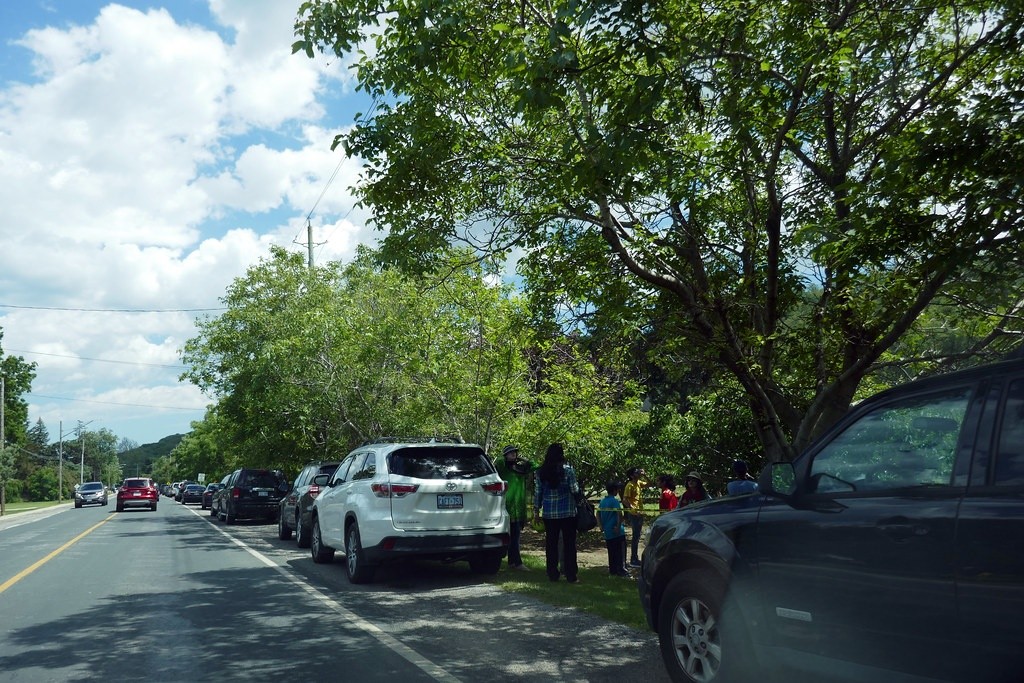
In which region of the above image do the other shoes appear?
[629,556,642,568]
[624,572,633,579]
[573,579,581,584]
[556,574,567,581]
[508,563,515,568]
[515,564,530,572]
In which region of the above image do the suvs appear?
[175,482,184,501]
[638,347,1024,683]
[217,467,290,525]
[177,481,199,502]
[154,483,159,501]
[309,437,510,585]
[117,477,157,511]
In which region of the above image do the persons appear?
[676,471,712,511]
[656,473,678,514]
[726,460,758,495]
[495,445,540,571]
[557,528,565,576]
[534,442,583,583]
[623,467,654,567]
[596,480,631,578]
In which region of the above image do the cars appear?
[162,486,170,495]
[160,484,167,492]
[201,483,219,510]
[277,460,342,548]
[166,488,172,497]
[182,485,207,505]
[211,474,231,516]
[75,482,108,508]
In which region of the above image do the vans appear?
[171,483,179,494]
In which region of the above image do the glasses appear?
[632,470,639,475]
[688,479,696,482]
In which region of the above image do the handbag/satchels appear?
[576,494,597,530]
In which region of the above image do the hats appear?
[606,481,623,491]
[503,445,518,455]
[687,471,701,480]
